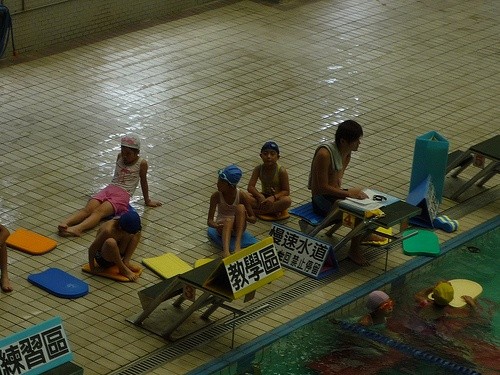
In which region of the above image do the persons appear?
[0,225,12,292]
[207,165,256,258]
[307,121,386,267]
[360,290,392,328]
[58,133,162,237]
[247,141,291,219]
[88,211,141,281]
[415,280,475,313]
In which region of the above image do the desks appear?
[444,135,500,200]
[308,201,422,253]
[136,257,234,337]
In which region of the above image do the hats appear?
[432,280,455,306]
[119,211,142,232]
[367,290,390,311]
[260,141,281,154]
[120,133,141,150]
[220,165,243,182]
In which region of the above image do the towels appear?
[307,142,344,190]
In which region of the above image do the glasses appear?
[217,169,235,187]
[262,142,278,148]
[377,300,394,310]
[436,280,451,286]
